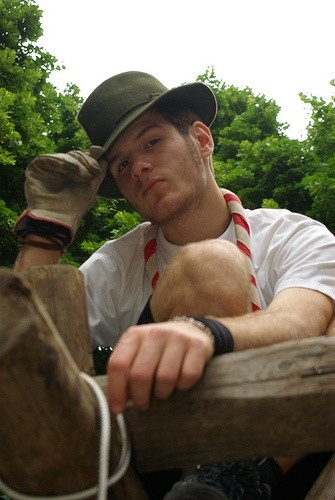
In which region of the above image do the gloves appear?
[13,145,107,248]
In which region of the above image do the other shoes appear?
[164,455,272,500]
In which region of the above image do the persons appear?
[14,71,335,500]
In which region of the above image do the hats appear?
[77,71,217,198]
